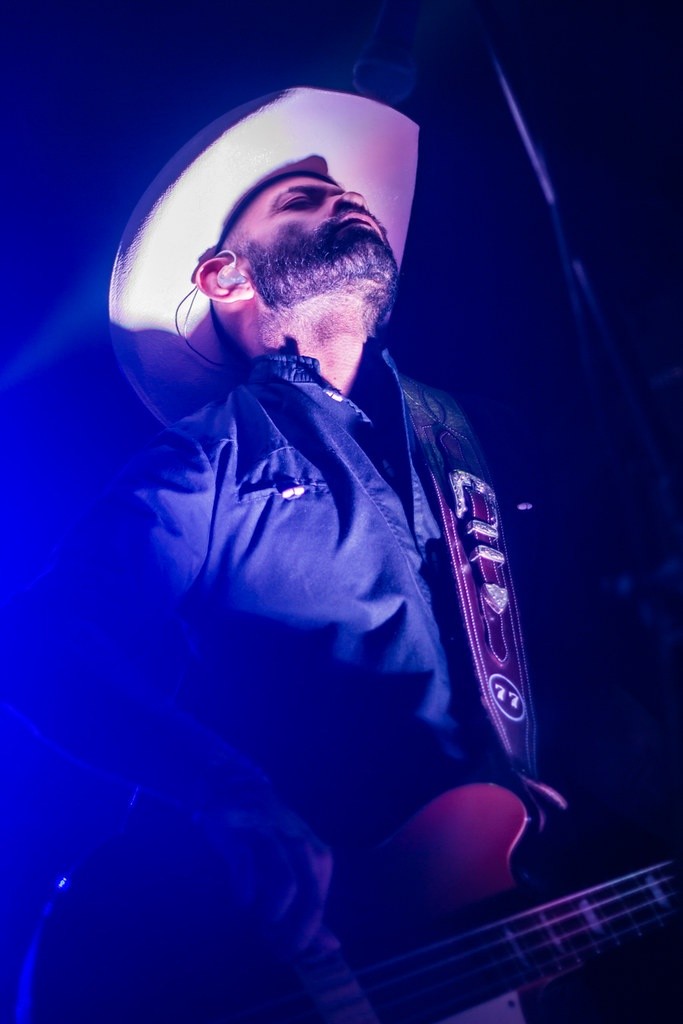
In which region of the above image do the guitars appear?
[15,782,682,1023]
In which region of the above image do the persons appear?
[0,83,574,1024]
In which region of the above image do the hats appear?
[104,88,422,425]
